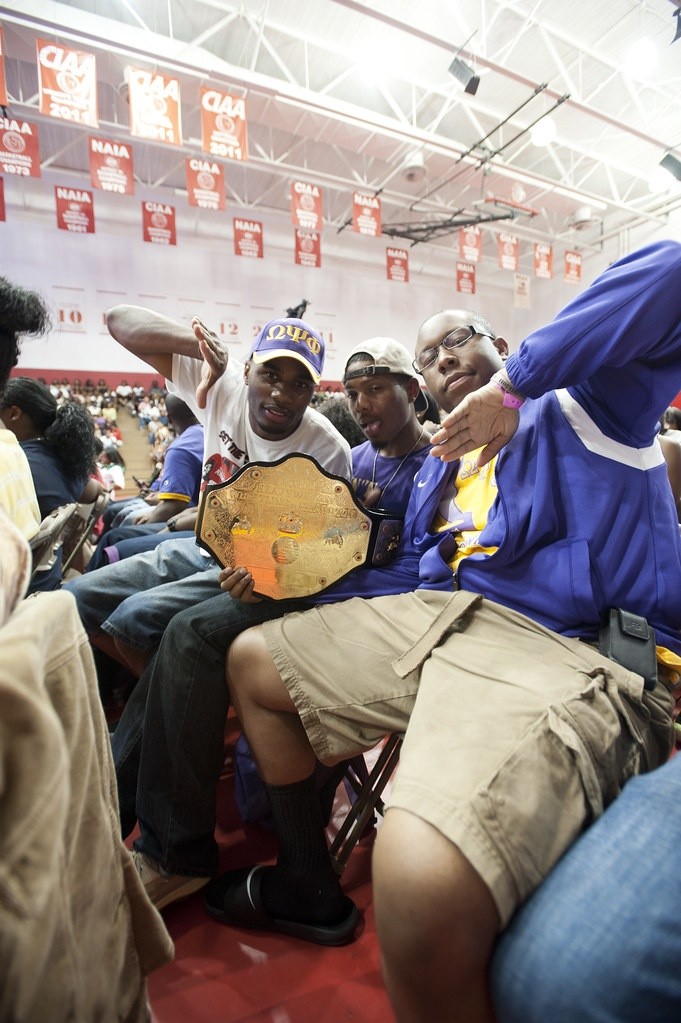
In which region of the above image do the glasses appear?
[412,324,496,374]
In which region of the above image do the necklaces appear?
[372,425,425,507]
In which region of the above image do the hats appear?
[342,338,429,412]
[248,318,325,385]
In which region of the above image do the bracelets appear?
[493,371,527,401]
[490,380,523,409]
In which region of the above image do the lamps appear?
[660,154,681,186]
[400,57,481,182]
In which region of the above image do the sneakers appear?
[132,851,208,910]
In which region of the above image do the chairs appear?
[26,479,114,591]
[327,732,407,875]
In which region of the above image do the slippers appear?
[203,866,357,946]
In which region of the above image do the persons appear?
[202,235,681,1023]
[0,274,681,911]
[489,745,681,1023]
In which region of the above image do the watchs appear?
[167,517,178,532]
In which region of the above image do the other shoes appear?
[111,676,136,699]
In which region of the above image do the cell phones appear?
[131,476,142,490]
